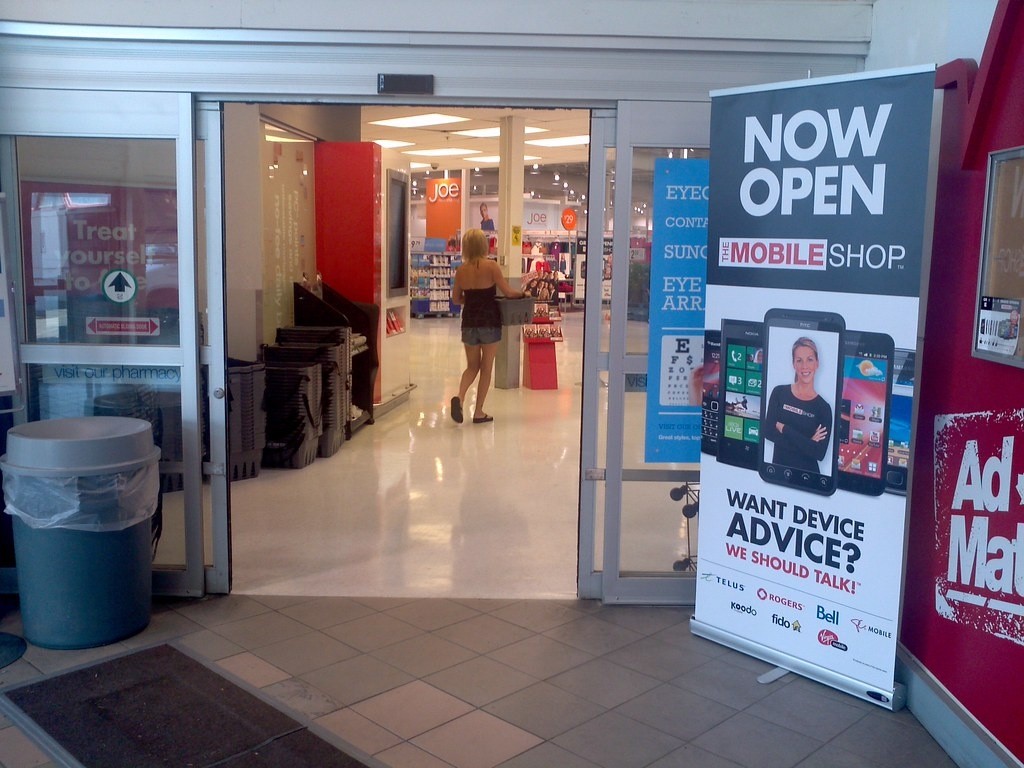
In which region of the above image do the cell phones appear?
[700,308,918,497]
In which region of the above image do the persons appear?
[765,337,833,473]
[451,229,532,423]
[480,203,495,231]
[527,271,558,305]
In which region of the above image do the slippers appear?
[450,397,463,423]
[473,414,493,423]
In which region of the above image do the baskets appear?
[90,326,352,492]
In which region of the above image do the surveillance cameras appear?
[431,163,440,170]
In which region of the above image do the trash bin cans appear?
[0,415,162,650]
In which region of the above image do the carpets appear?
[0,639,394,768]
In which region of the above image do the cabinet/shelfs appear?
[298,283,378,438]
[411,250,461,318]
